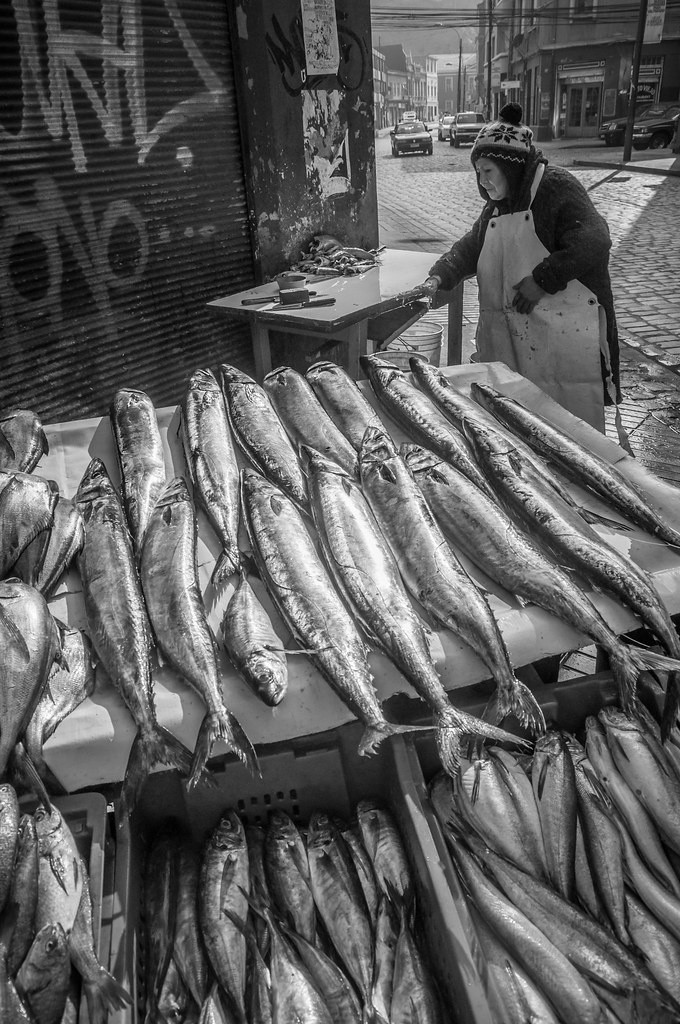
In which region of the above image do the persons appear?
[420,100,622,437]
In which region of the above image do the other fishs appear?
[0,351,679,1024]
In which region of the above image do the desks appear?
[205,248,477,381]
[25,360,680,792]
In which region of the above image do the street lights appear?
[446,63,466,112]
[433,23,462,114]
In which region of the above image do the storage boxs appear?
[19,664,680,1024]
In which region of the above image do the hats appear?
[470,103,533,216]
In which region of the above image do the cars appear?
[437,116,456,142]
[599,101,680,148]
[389,121,434,158]
[632,104,680,150]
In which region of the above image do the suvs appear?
[449,111,488,149]
